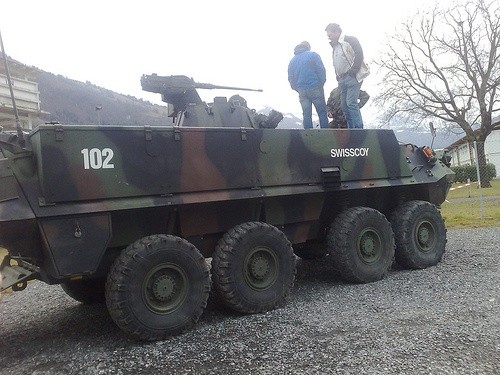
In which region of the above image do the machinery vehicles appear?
[0,72,458,343]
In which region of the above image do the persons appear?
[324,23,371,129]
[441,148,452,168]
[287,40,329,129]
[326,76,370,128]
